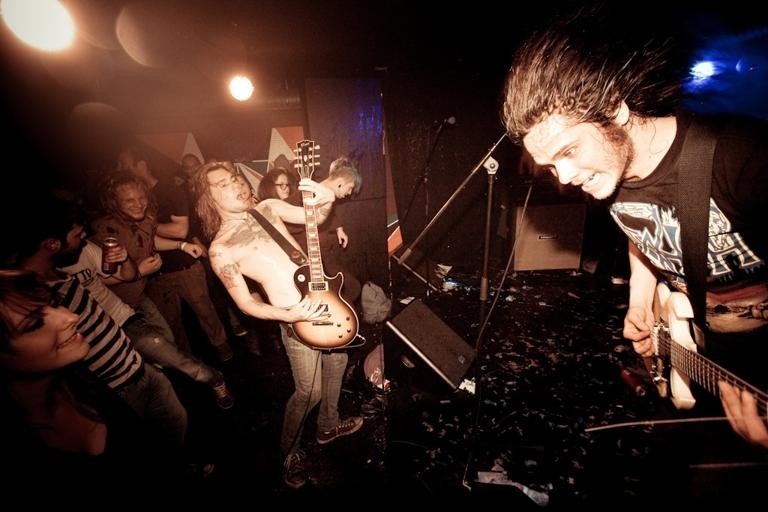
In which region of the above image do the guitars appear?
[640,283,766,423]
[292,139,359,350]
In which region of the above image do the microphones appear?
[425,116,456,131]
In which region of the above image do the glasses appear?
[274,184,288,190]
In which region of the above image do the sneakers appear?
[232,325,247,336]
[219,342,233,361]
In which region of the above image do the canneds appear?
[102,237,120,274]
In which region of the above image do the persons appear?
[502,31,768,448]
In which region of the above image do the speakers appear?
[513,204,586,272]
[386,298,476,390]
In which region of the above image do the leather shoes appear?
[212,372,233,409]
[284,450,306,487]
[316,416,363,444]
